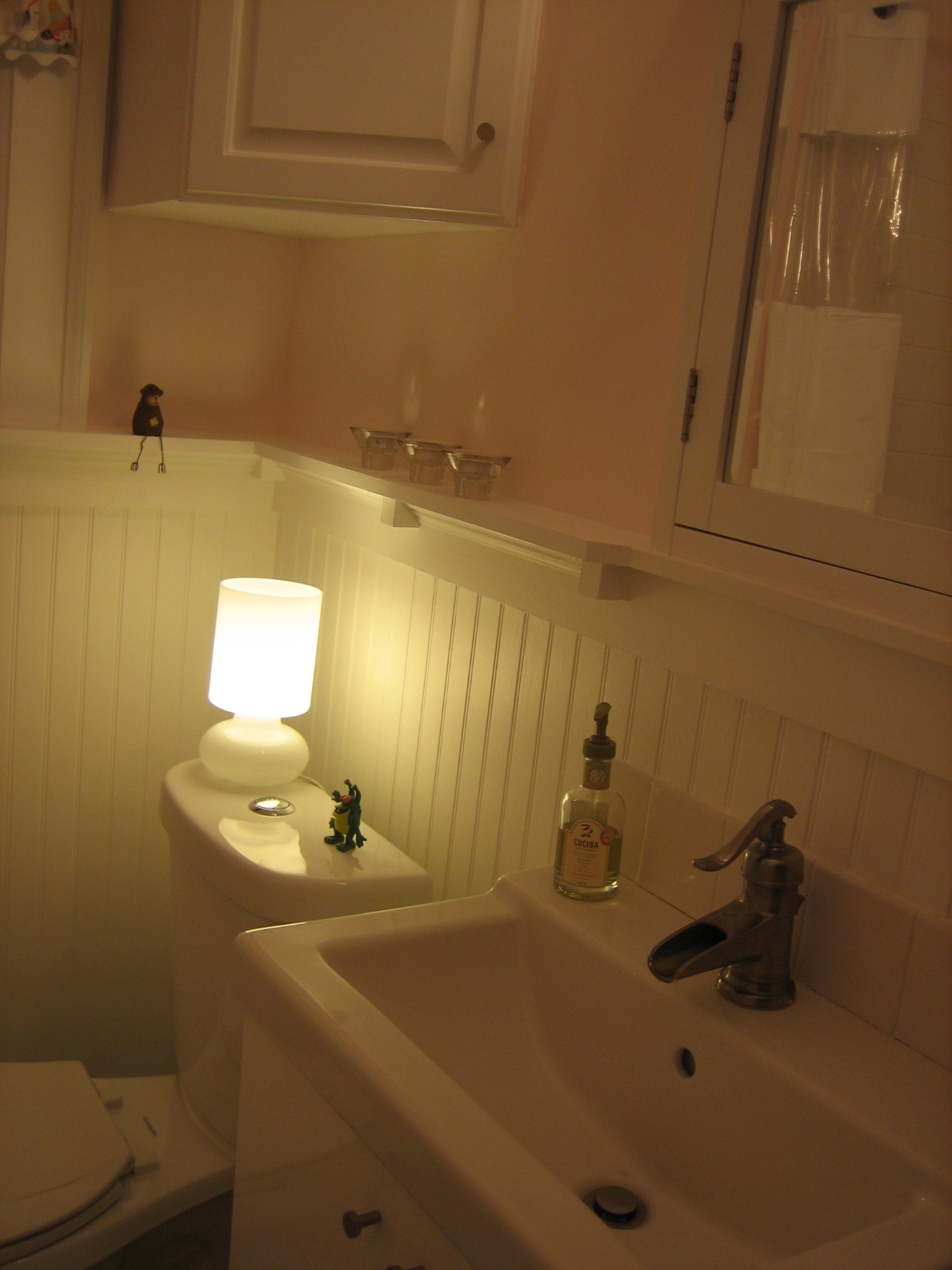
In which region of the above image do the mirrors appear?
[722,1,952,530]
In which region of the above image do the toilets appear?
[1,756,433,1269]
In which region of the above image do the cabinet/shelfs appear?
[105,1,544,240]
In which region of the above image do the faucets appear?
[646,798,806,1011]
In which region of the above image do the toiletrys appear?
[551,702,627,901]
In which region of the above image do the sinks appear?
[234,875,952,1270]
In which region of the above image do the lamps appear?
[199,579,325,787]
